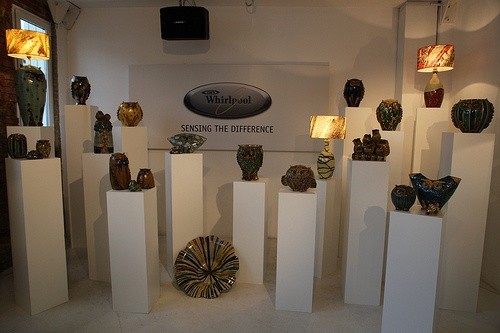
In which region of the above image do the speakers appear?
[159,6,210,40]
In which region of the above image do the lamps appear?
[6,29,50,126]
[309,115,346,180]
[416,45,455,108]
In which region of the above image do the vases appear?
[237,144,263,181]
[70,75,91,105]
[117,102,143,126]
[36,139,51,159]
[451,98,495,133]
